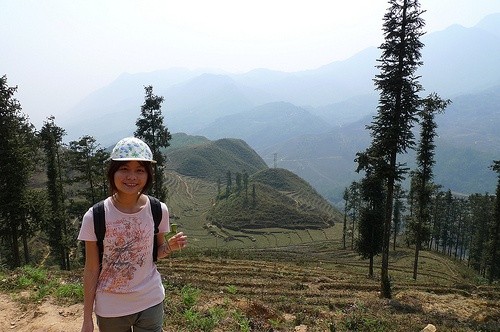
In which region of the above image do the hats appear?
[103,138,159,165]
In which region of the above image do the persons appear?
[76,136,187,332]
